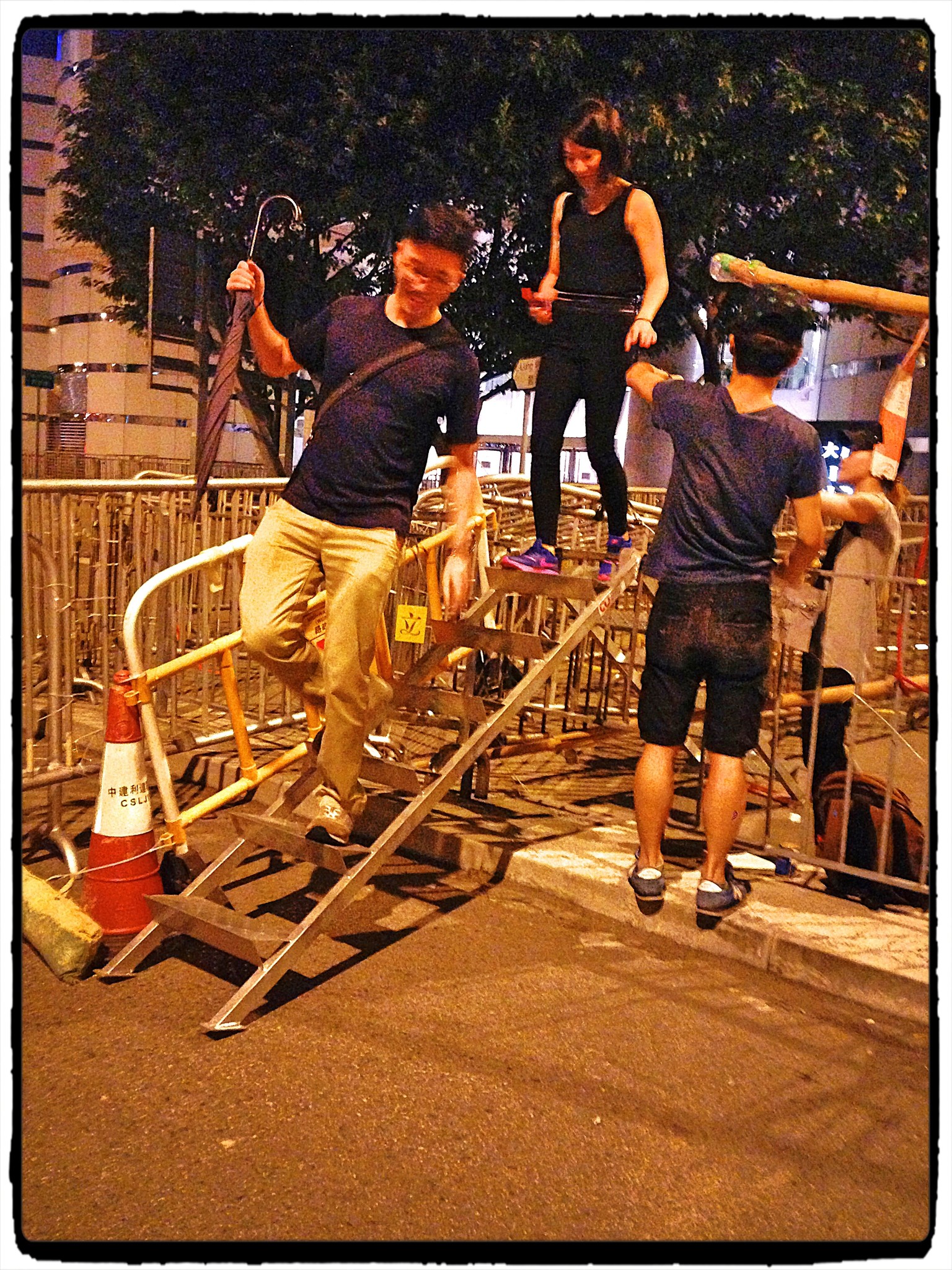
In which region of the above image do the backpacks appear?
[815,771,927,907]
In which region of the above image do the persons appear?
[223,200,489,845]
[781,431,916,848]
[615,310,828,920]
[491,100,671,587]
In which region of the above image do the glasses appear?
[395,258,461,292]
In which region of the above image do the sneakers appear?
[501,538,559,576]
[305,795,353,846]
[696,872,752,917]
[628,861,666,900]
[597,536,632,581]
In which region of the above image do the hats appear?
[837,423,912,460]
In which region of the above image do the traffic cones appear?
[78,672,169,961]
